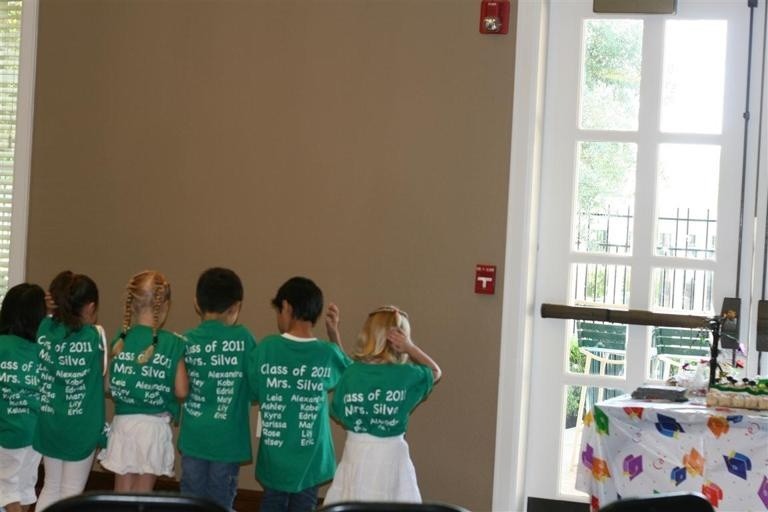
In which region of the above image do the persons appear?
[324,306,441,505]
[1,285,48,512]
[95,272,187,495]
[182,267,261,505]
[252,277,349,510]
[34,270,114,512]
